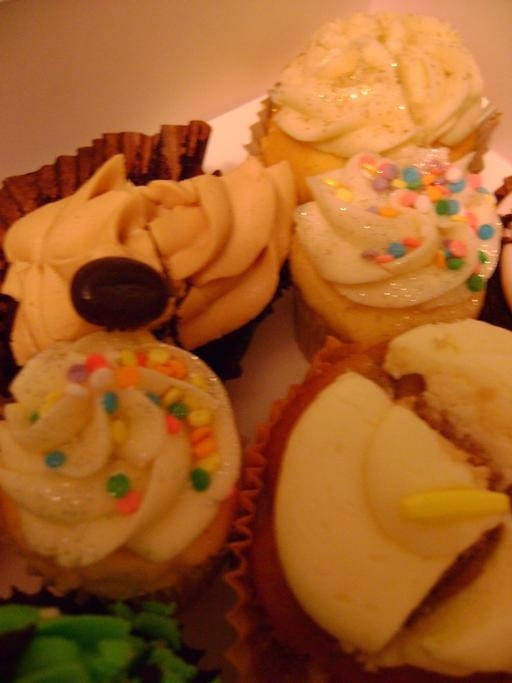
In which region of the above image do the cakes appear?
[227,319,511,679]
[0,598,217,681]
[1,151,295,373]
[1,332,242,596]
[293,146,502,341]
[247,14,500,201]
